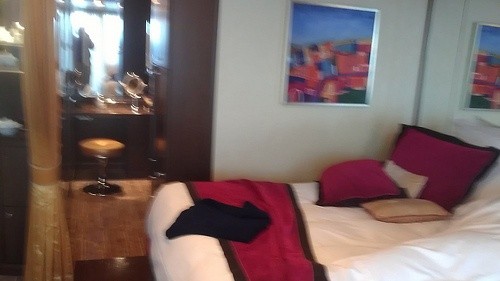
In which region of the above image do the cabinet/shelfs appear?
[75,102,154,168]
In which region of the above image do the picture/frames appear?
[281,0,381,109]
[459,23,500,111]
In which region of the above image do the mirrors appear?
[55,0,128,97]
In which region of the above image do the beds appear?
[140,116,500,281]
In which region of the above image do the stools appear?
[82,139,124,196]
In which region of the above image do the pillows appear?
[315,127,500,224]
